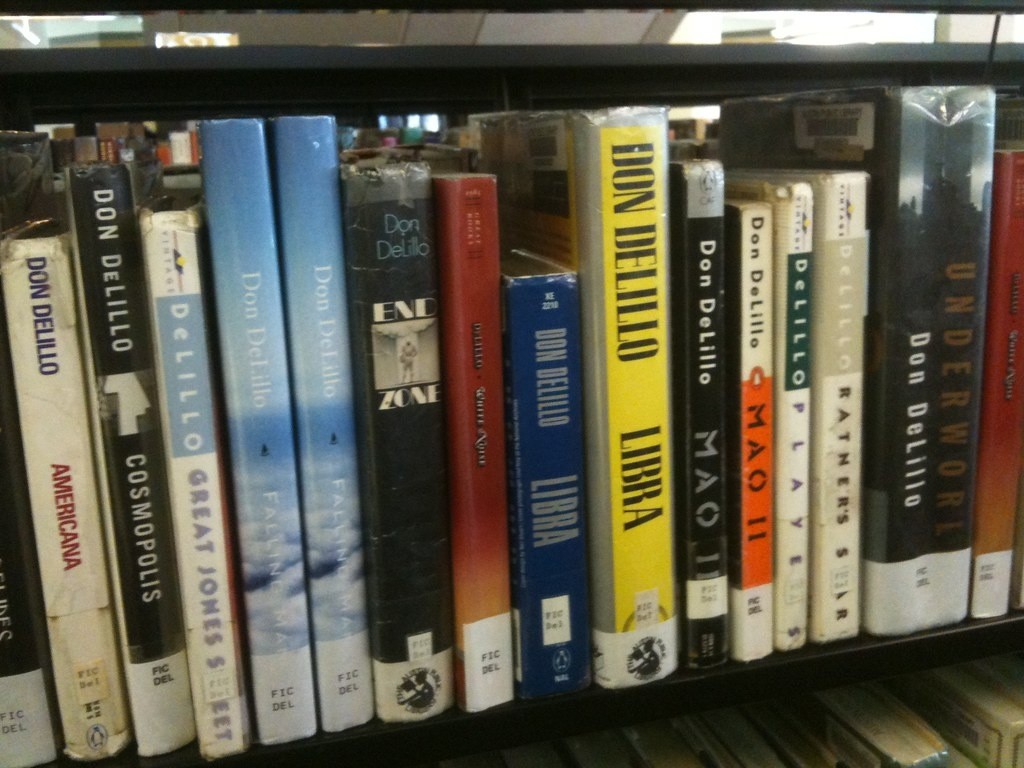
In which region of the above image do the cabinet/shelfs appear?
[0,0,1024,768]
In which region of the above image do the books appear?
[722,197,775,661]
[500,247,592,697]
[141,188,251,763]
[0,84,1024,768]
